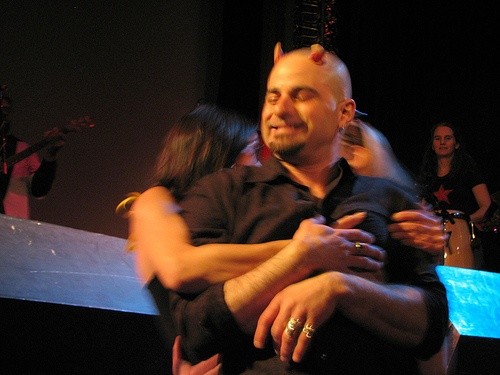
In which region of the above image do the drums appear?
[433,209,476,269]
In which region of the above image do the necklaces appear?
[437,161,452,173]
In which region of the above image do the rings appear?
[352,243,362,254]
[303,325,316,338]
[281,317,303,338]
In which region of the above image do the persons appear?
[1,83,66,224]
[417,125,492,269]
[165,41,450,375]
[125,103,448,374]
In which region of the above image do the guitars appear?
[0,113,97,174]
